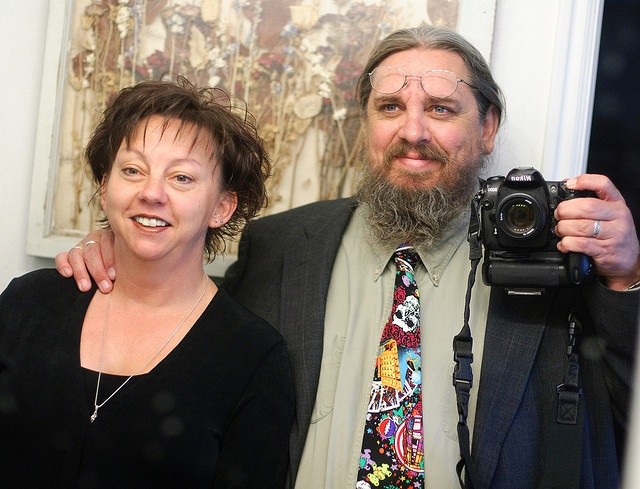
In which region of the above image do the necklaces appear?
[89,273,211,423]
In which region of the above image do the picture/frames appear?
[25,2,495,277]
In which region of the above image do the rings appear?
[591,219,600,238]
[67,246,83,253]
[84,240,97,248]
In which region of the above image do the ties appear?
[356,242,424,489]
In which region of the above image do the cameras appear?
[467,166,594,305]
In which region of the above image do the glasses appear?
[365,65,478,98]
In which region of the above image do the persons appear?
[52,27,639,485]
[0,76,293,489]
